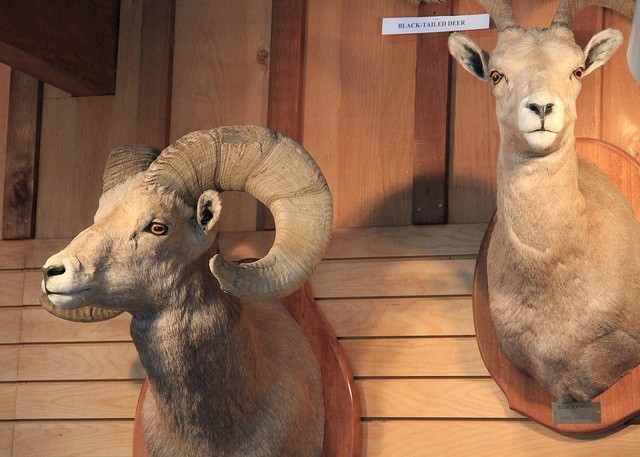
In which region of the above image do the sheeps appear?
[41,124,333,456]
[446,0,640,405]
